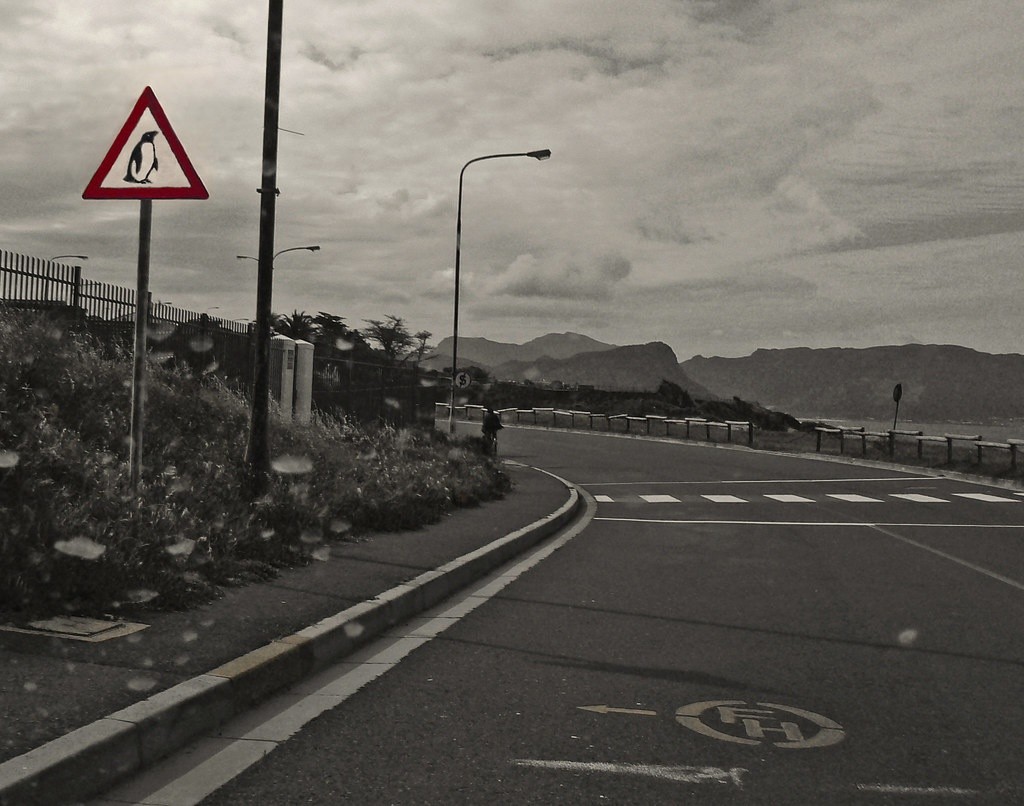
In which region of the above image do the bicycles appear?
[484,431,499,455]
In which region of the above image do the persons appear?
[481,408,504,453]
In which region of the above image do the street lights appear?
[448,148,551,433]
[205,306,219,313]
[236,245,321,261]
[158,301,172,305]
[234,318,249,322]
[48,255,89,264]
[77,283,102,286]
